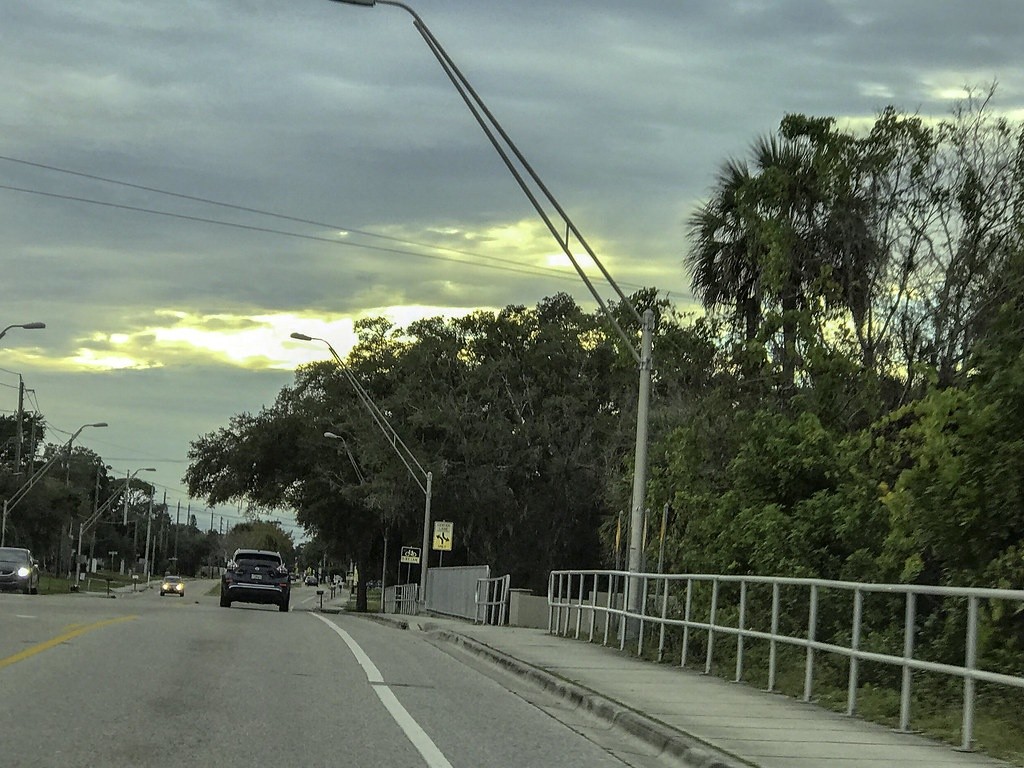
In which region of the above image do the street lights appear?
[2,423,111,550]
[74,467,156,588]
[321,431,392,614]
[290,330,434,609]
[330,1,657,641]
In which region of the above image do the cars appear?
[290,573,296,581]
[219,548,290,613]
[0,547,40,595]
[304,576,319,587]
[160,576,185,597]
